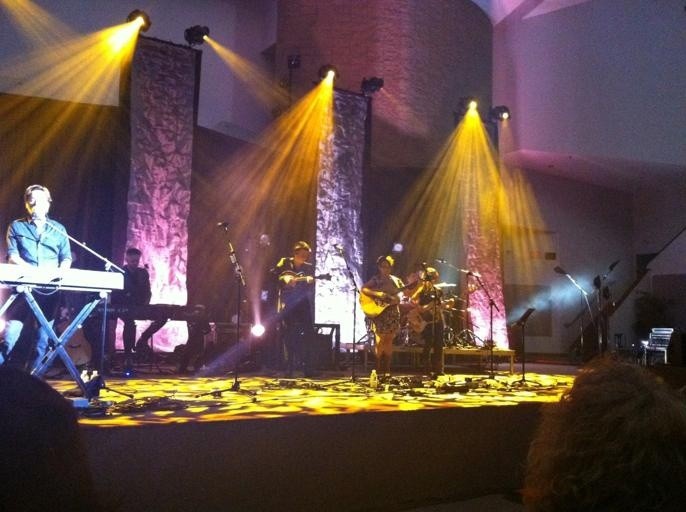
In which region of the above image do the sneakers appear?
[304,373,319,377]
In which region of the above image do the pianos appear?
[87,304,197,320]
[0,263,124,294]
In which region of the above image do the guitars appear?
[407,288,478,333]
[278,271,332,289]
[359,280,423,317]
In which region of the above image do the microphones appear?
[217,221,229,227]
[31,210,39,219]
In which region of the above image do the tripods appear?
[450,264,485,346]
[194,258,256,399]
[321,284,367,388]
[509,332,543,386]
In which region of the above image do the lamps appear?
[360,77,384,95]
[127,9,151,32]
[185,25,210,45]
[319,63,340,84]
[491,104,511,122]
[459,95,479,114]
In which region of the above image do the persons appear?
[522,354,686,511]
[361,256,455,376]
[109,248,167,355]
[271,242,314,379]
[1,367,89,512]
[0,185,72,373]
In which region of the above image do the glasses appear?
[131,260,139,263]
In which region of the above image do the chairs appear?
[640,328,674,366]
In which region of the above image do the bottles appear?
[370,370,377,388]
